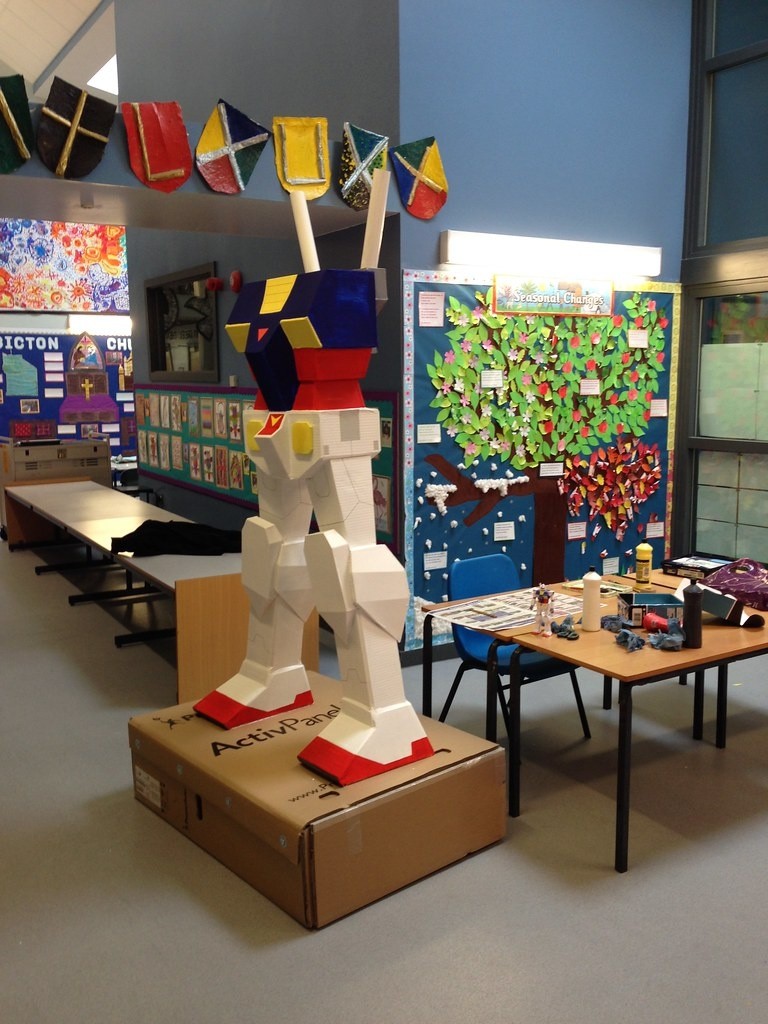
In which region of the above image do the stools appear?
[116,485,153,503]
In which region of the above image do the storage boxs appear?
[617,592,684,626]
[129,672,509,929]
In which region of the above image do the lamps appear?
[440,229,662,276]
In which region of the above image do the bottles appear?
[635,538,654,588]
[682,578,702,648]
[582,566,601,632]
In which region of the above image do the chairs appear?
[438,554,591,764]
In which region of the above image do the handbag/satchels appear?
[696,557,768,611]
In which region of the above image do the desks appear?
[422,569,768,872]
[3,476,319,705]
